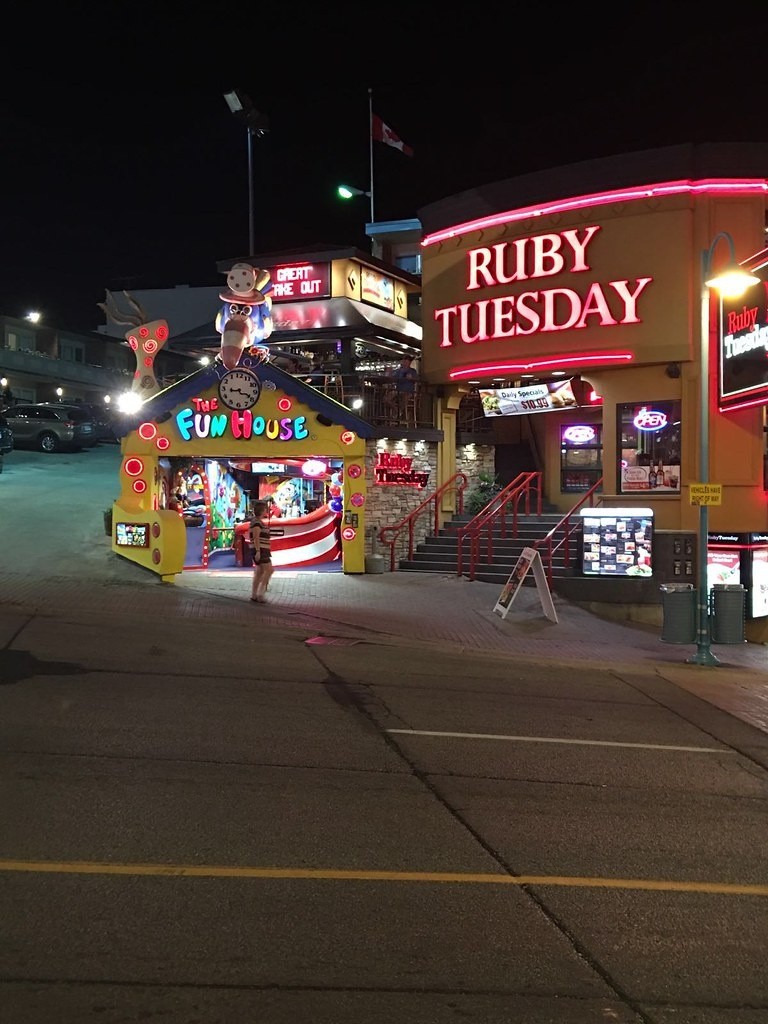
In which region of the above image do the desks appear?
[359,376,392,425]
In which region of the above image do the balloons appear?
[331,473,341,486]
[329,484,341,497]
[328,499,342,513]
[338,471,343,483]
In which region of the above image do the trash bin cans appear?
[659,583,698,644]
[710,584,748,644]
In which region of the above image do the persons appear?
[663,446,680,466]
[286,357,301,374]
[303,355,324,387]
[382,355,418,419]
[249,500,274,604]
[263,496,281,519]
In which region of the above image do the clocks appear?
[218,368,262,411]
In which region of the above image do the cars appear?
[76,403,127,445]
[0,415,13,455]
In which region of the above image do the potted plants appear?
[467,471,513,516]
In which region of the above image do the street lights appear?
[223,89,254,255]
[684,230,761,667]
[337,185,374,224]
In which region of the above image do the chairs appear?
[389,382,418,429]
[324,375,362,416]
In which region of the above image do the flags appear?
[372,113,414,158]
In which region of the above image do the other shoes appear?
[256,601,267,604]
[250,598,258,602]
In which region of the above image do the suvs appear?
[0,404,94,453]
